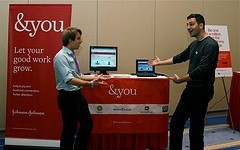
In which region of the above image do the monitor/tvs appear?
[89,46,117,71]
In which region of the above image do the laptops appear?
[136,59,157,77]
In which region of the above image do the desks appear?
[227,71,240,130]
[80,72,171,149]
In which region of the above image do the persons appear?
[53,26,110,150]
[147,13,219,150]
